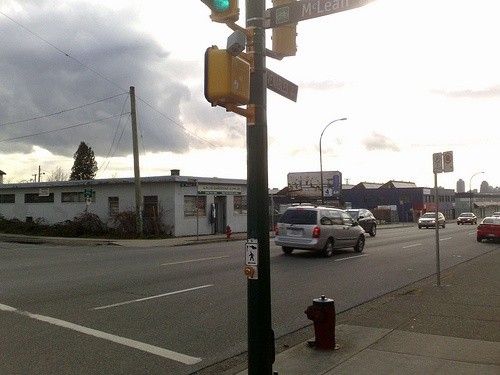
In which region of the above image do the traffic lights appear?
[208,0,239,23]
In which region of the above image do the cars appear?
[274,205,366,258]
[476,217,500,242]
[344,209,377,237]
[418,212,446,229]
[457,213,477,225]
[491,212,500,217]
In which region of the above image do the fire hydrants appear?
[304,295,336,350]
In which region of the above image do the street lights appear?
[470,171,485,213]
[319,118,348,205]
